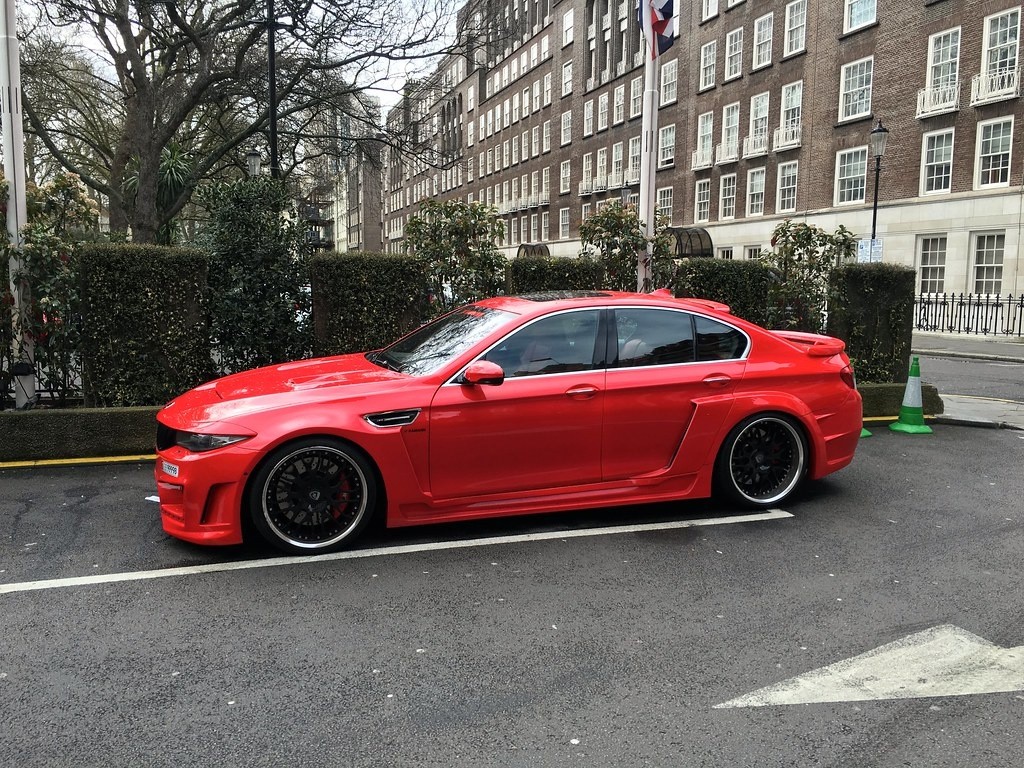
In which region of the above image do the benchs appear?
[619,339,693,365]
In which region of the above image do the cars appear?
[153,288,862,558]
[428,284,490,314]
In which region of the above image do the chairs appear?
[570,324,596,364]
[519,317,571,372]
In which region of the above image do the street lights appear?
[857,118,890,263]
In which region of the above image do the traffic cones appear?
[888,356,934,434]
[849,358,872,438]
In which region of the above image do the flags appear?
[639,0,674,61]
